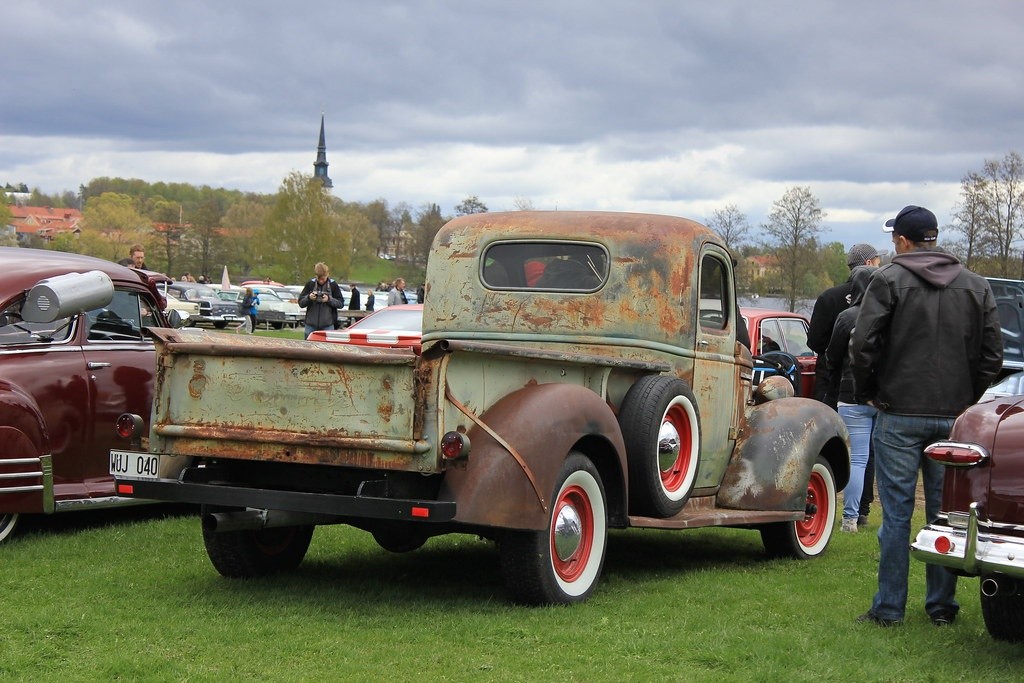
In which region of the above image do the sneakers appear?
[839,515,859,533]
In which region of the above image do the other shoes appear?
[856,609,902,628]
[857,515,867,525]
[932,611,954,626]
[236,328,239,334]
[248,333,253,334]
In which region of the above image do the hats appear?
[253,289,259,294]
[847,244,886,270]
[883,205,938,242]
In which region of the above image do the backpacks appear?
[752,351,802,398]
[307,281,337,322]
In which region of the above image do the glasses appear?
[134,256,146,260]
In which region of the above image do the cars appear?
[1,209,1024,648]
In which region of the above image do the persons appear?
[848,205,1005,626]
[109,244,261,335]
[536,247,608,289]
[344,277,426,331]
[299,262,346,342]
[806,243,886,532]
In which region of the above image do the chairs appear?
[485,257,511,286]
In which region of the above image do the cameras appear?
[314,290,325,302]
